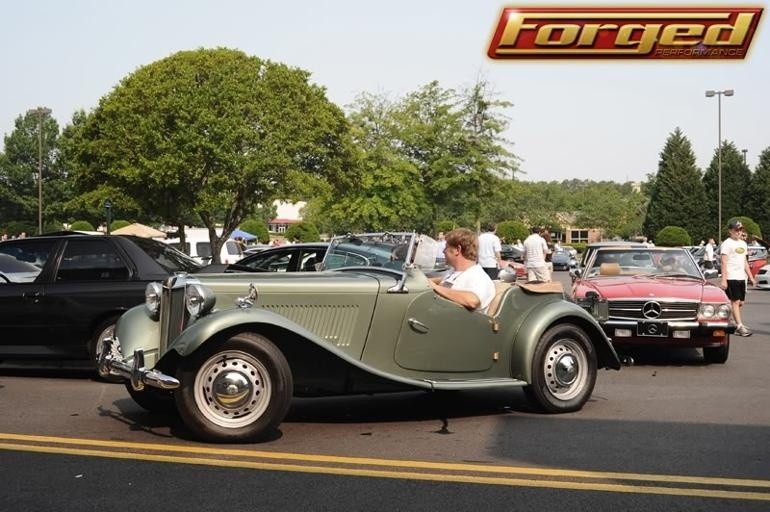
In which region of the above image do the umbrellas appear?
[109,223,166,238]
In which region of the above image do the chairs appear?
[600,263,621,275]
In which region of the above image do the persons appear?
[0,232,26,242]
[234,234,303,254]
[430,222,749,315]
[719,222,756,337]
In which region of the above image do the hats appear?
[728,220,744,231]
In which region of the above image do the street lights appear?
[30,108,51,236]
[103,197,115,236]
[705,90,734,244]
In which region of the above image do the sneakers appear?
[734,325,753,338]
